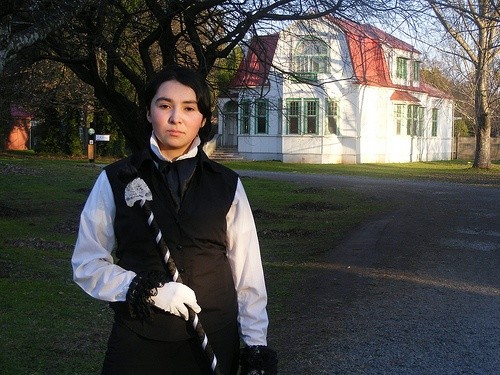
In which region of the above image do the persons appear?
[71,64,280,375]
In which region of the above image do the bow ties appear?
[149,147,199,213]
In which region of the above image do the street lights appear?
[30,121,38,150]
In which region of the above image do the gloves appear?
[125,265,201,321]
[238,345,279,375]
